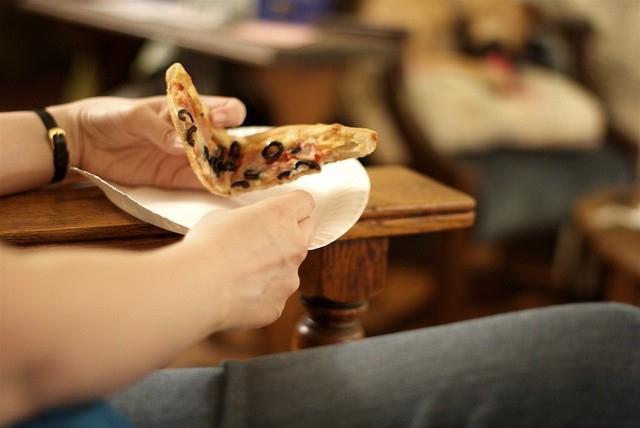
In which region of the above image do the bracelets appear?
[35,103,68,186]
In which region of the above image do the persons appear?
[1,95,621,347]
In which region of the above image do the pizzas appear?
[164,63,378,198]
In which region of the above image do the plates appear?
[64,125,372,253]
